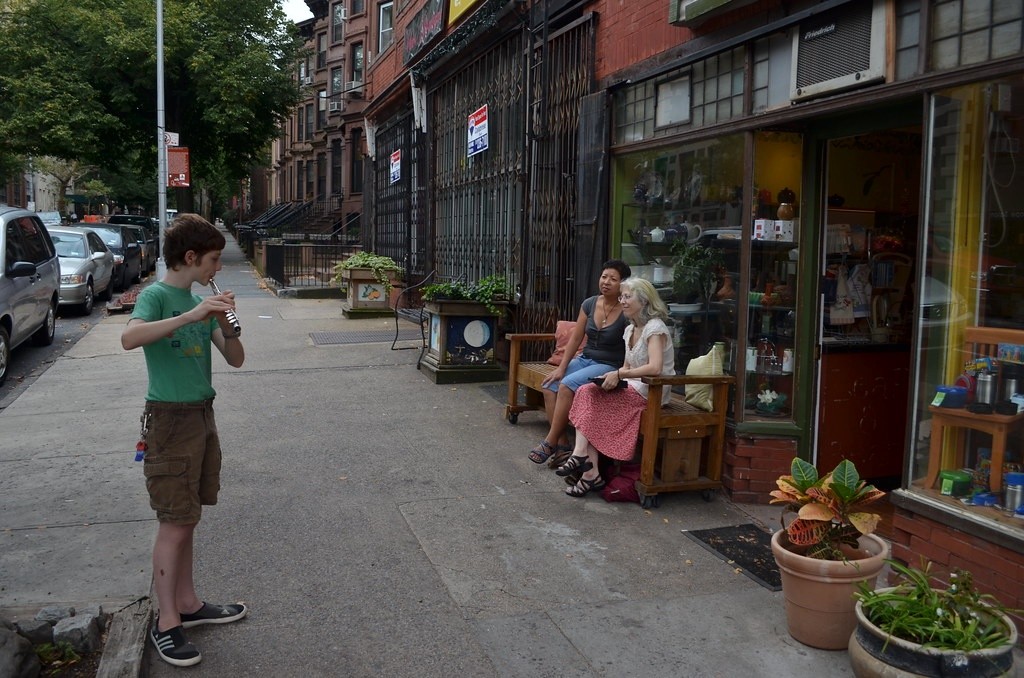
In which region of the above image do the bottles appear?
[759,188,772,203]
[776,203,793,220]
[778,187,796,203]
[760,283,776,306]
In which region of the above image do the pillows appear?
[547,320,588,366]
[683,340,725,412]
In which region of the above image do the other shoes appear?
[565,474,586,486]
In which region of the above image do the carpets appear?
[681,523,782,592]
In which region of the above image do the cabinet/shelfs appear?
[654,238,799,420]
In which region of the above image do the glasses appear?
[617,294,640,302]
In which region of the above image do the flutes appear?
[208,275,242,334]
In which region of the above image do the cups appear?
[782,348,794,372]
[1006,484,1022,510]
[972,373,1019,405]
[746,347,758,371]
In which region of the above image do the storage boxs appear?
[754,220,795,242]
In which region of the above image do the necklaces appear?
[602,301,619,323]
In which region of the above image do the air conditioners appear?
[305,77,313,84]
[341,8,347,21]
[328,102,340,112]
[345,80,361,93]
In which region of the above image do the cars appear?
[35,210,66,226]
[68,213,174,292]
[824,226,1024,352]
[46,226,115,317]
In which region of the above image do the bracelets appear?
[618,370,620,380]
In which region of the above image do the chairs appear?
[924,326,1024,507]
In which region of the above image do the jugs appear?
[649,221,703,244]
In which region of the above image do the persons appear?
[528,259,630,469]
[70,211,77,223]
[121,215,247,665]
[555,277,676,498]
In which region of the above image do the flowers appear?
[851,553,1024,678]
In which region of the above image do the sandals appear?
[528,440,558,464]
[556,455,594,477]
[548,445,573,469]
[565,473,606,497]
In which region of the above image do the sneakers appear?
[178,601,248,627]
[149,615,201,667]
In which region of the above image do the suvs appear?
[0,202,61,386]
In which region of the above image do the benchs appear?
[502,332,734,509]
[390,270,468,369]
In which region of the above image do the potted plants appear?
[746,381,789,417]
[331,251,406,319]
[768,457,889,650]
[418,273,514,384]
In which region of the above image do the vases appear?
[760,275,796,307]
[715,273,736,301]
[848,586,1018,678]
